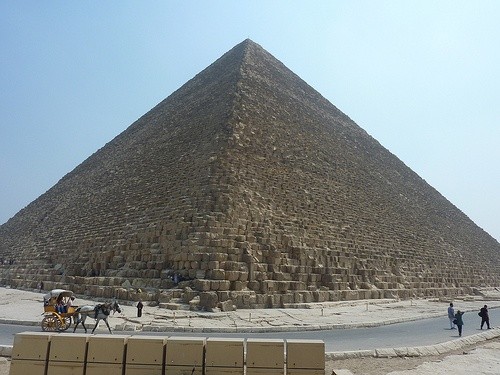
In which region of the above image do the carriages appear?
[39,289,122,334]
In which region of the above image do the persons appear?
[454,310,464,337]
[137,301,143,317]
[53,297,67,314]
[67,296,81,325]
[480,304,491,329]
[448,302,456,329]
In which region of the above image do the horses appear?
[71,298,123,335]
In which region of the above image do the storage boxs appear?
[8,331,327,375]
